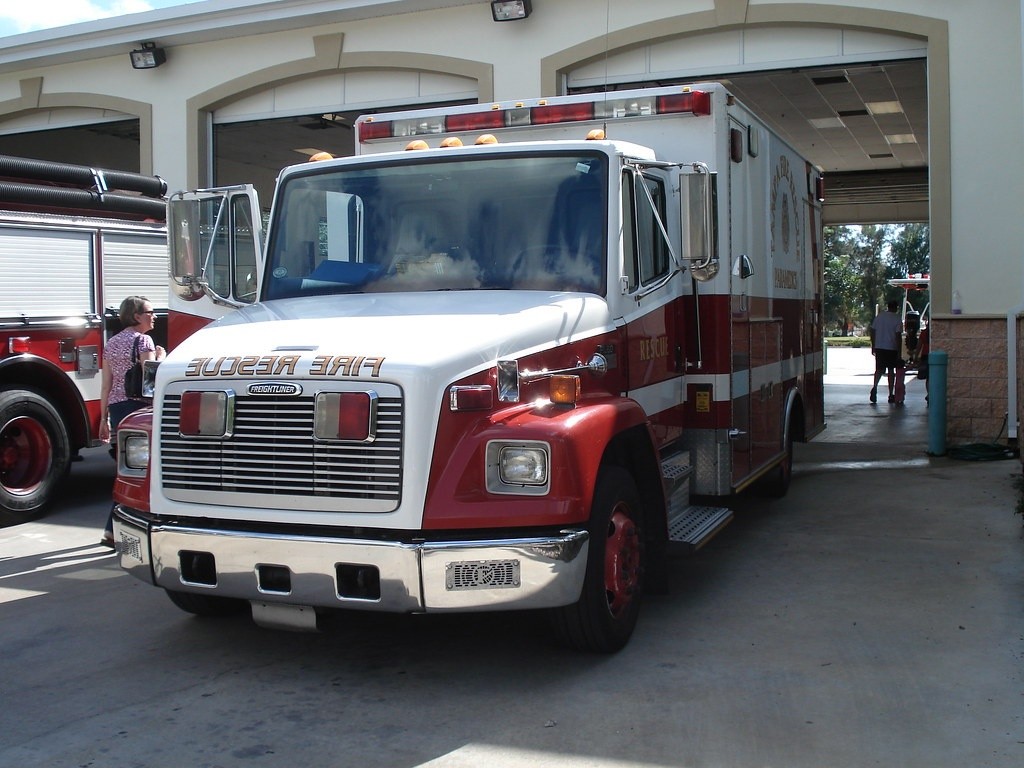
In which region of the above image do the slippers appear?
[99,538,114,549]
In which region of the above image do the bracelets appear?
[100,418,107,420]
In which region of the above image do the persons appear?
[870,301,929,406]
[99,295,165,548]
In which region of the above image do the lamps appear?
[490,0,531,21]
[129,40,167,69]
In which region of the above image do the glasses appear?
[138,311,153,315]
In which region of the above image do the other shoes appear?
[888,395,896,403]
[870,387,876,403]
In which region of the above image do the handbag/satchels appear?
[125,335,152,399]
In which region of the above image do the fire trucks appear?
[873,272,929,371]
[111,80,827,656]
[0,155,173,518]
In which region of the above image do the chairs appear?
[389,206,459,271]
